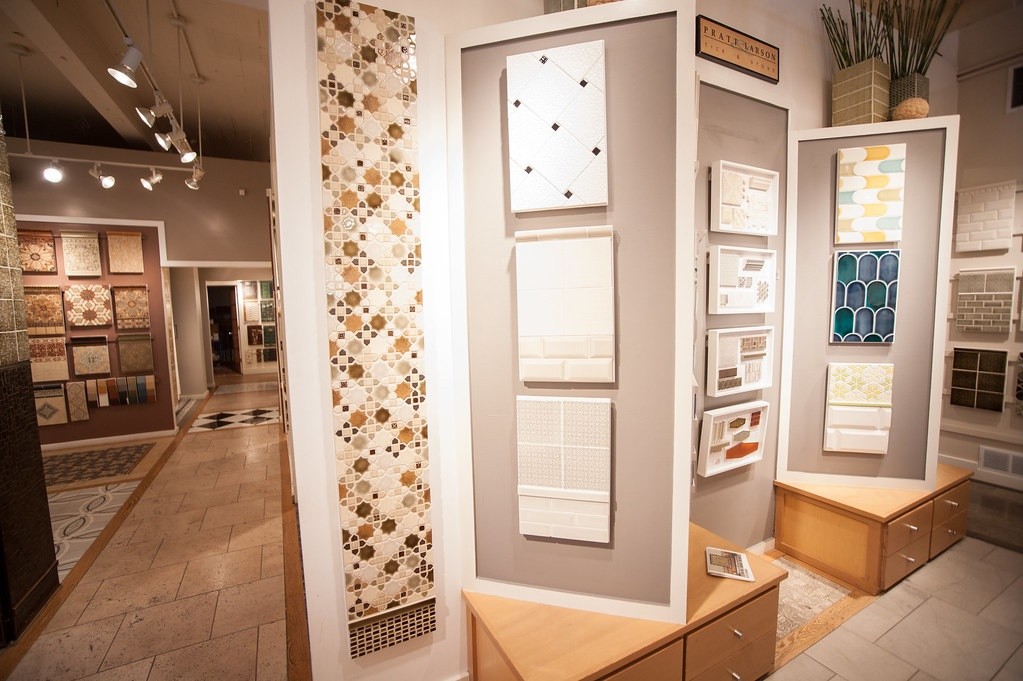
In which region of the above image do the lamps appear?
[42,37,203,191]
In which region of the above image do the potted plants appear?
[817,1,963,128]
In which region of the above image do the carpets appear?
[42,442,158,487]
[760,550,878,680]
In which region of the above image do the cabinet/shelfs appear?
[465,521,788,681]
[773,463,976,595]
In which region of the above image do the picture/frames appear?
[706,325,774,398]
[696,14,779,85]
[708,245,776,315]
[696,400,770,478]
[711,160,780,237]
